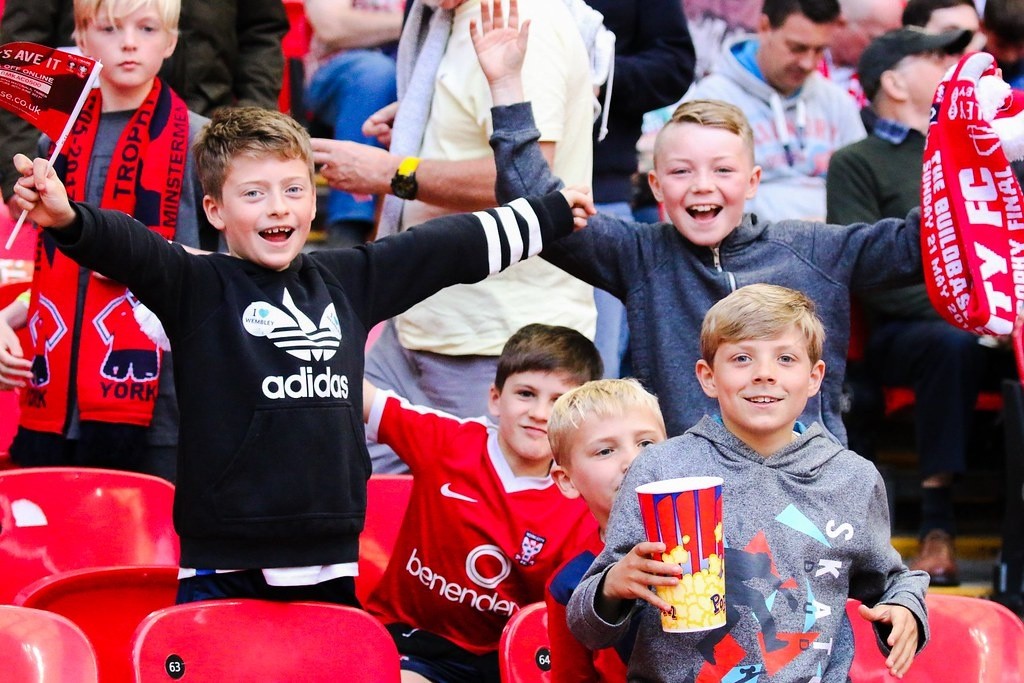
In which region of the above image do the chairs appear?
[0,467,1024,683]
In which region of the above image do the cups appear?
[635,477,727,633]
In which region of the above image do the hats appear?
[856,28,973,101]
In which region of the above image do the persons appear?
[0,0,1024,619]
[564,283,931,683]
[364,324,608,683]
[12,106,599,610]
[550,377,669,683]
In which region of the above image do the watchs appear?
[390,157,422,201]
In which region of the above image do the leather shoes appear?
[909,528,959,587]
